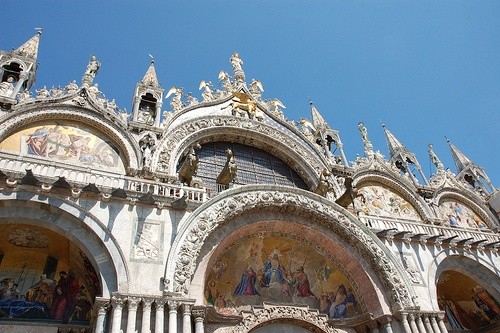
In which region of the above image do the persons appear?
[164,51,328,152]
[331,140,467,194]
[1,45,163,144]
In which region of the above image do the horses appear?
[314,167,338,199]
[335,176,366,210]
[216,148,238,191]
[178,142,201,186]
